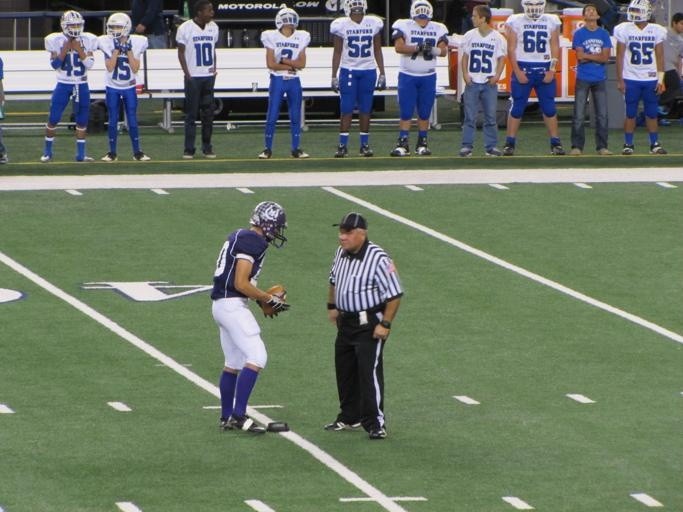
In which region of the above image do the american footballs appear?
[259,286,287,315]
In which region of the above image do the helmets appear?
[624,2,654,22]
[273,7,302,30]
[59,10,85,39]
[521,0,548,20]
[409,2,436,22]
[344,1,370,18]
[248,200,291,249]
[106,13,134,39]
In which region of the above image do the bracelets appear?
[550,58,559,73]
[656,72,665,84]
[280,57,283,63]
[327,303,336,309]
[381,320,390,329]
[431,47,441,56]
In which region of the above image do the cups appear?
[251,82,258,92]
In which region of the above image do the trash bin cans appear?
[589,57,626,128]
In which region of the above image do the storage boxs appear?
[488,3,589,99]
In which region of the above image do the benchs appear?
[0,48,455,131]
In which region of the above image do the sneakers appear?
[457,144,671,158]
[325,420,362,432]
[414,144,431,155]
[259,149,272,159]
[391,143,410,156]
[225,415,266,433]
[291,149,309,159]
[38,149,220,165]
[368,427,387,439]
[334,147,348,158]
[359,146,375,157]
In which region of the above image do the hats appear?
[331,211,369,231]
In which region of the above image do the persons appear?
[390,0,449,157]
[175,0,220,158]
[329,0,386,157]
[131,0,169,49]
[659,12,683,126]
[40,10,98,162]
[613,0,669,154]
[503,0,566,155]
[571,5,613,155]
[211,201,290,433]
[98,12,151,161]
[459,5,507,157]
[257,8,310,158]
[0,57,8,163]
[324,211,404,439]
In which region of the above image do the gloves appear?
[256,296,290,319]
[332,77,341,93]
[377,75,387,90]
[417,41,432,53]
